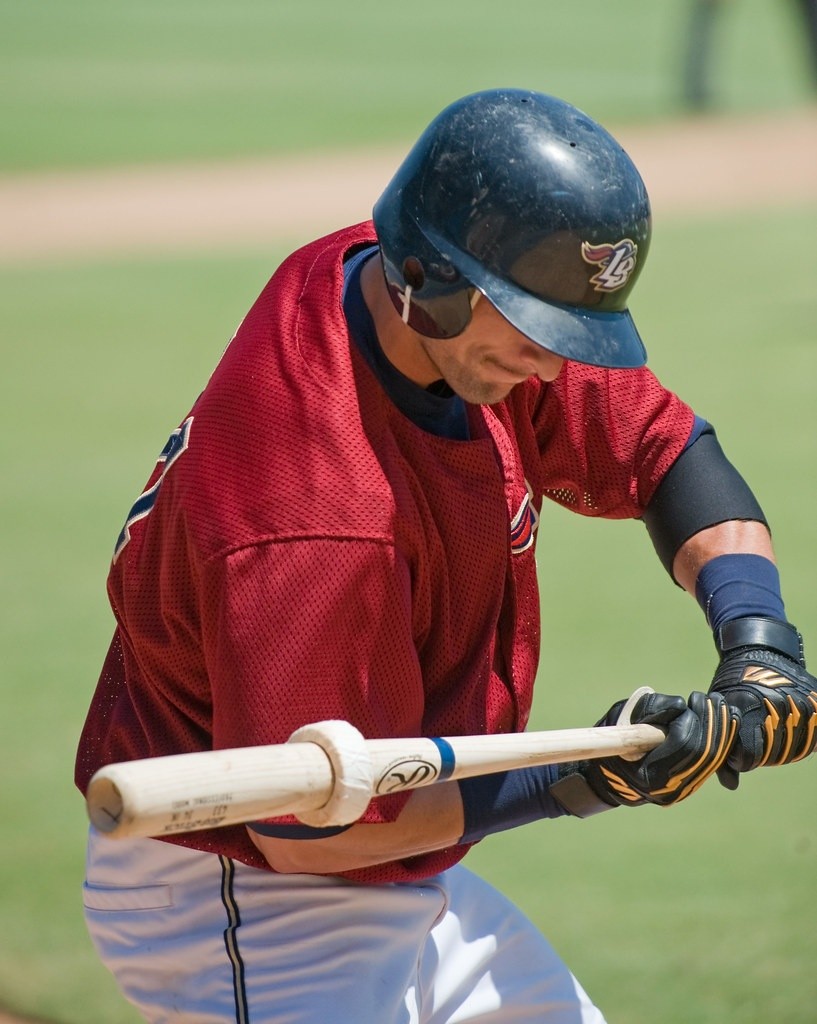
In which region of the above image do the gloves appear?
[708,617,817,790]
[584,691,742,808]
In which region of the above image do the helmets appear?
[372,89,652,369]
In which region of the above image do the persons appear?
[75,90,816,1024]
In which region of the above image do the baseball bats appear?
[86,721,665,841]
